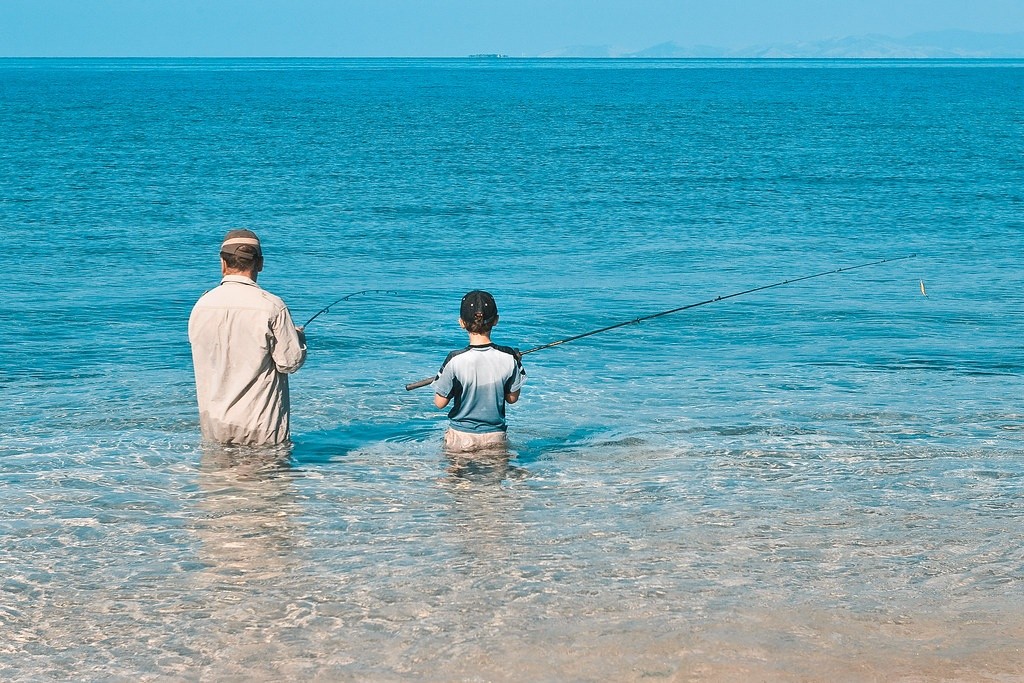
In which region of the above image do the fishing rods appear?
[406,253,917,391]
[298,289,398,330]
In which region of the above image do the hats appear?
[460,289,497,325]
[221,229,262,260]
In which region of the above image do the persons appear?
[188,228,308,446]
[430,292,528,448]
